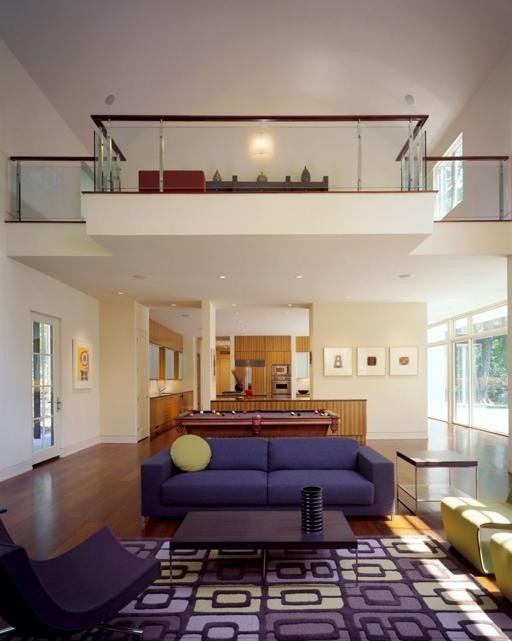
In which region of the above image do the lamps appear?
[249,130,274,159]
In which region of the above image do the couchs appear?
[138,170,206,194]
[141,437,394,516]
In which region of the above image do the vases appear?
[301,166,311,182]
[257,171,267,183]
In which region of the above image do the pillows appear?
[171,434,211,471]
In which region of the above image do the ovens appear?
[272,380,291,399]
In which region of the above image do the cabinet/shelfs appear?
[151,390,194,439]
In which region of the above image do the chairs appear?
[0,518,161,640]
[441,472,512,575]
[490,533,512,602]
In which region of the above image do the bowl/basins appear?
[298,390,309,394]
[244,390,255,395]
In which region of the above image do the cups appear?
[299,484,323,536]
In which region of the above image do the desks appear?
[175,409,339,438]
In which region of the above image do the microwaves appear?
[272,366,291,378]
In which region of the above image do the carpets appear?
[71,537,512,641]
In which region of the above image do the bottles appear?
[212,166,310,185]
